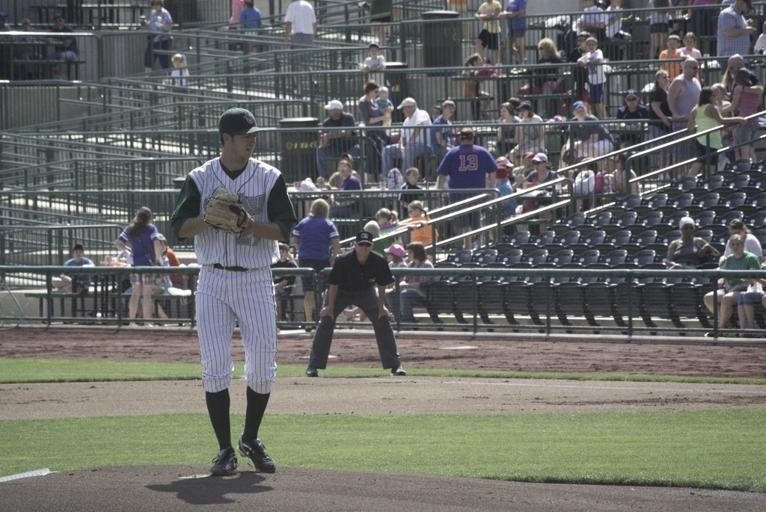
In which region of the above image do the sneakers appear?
[211,446,237,474]
[391,368,406,375]
[306,367,317,375]
[238,434,275,473]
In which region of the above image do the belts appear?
[213,262,250,271]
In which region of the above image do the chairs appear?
[432,154,765,338]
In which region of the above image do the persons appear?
[306,232,406,377]
[168,107,297,475]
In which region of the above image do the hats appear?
[679,217,696,230]
[356,232,373,245]
[324,100,344,110]
[397,97,417,110]
[384,244,406,258]
[532,153,549,163]
[496,156,515,167]
[363,220,380,234]
[625,89,641,99]
[219,108,268,135]
[571,101,585,112]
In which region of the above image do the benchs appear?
[289,159,592,222]
[288,32,720,158]
[23,288,305,330]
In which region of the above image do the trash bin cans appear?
[384,61,409,123]
[420,9,460,76]
[173,177,195,249]
[278,117,320,184]
